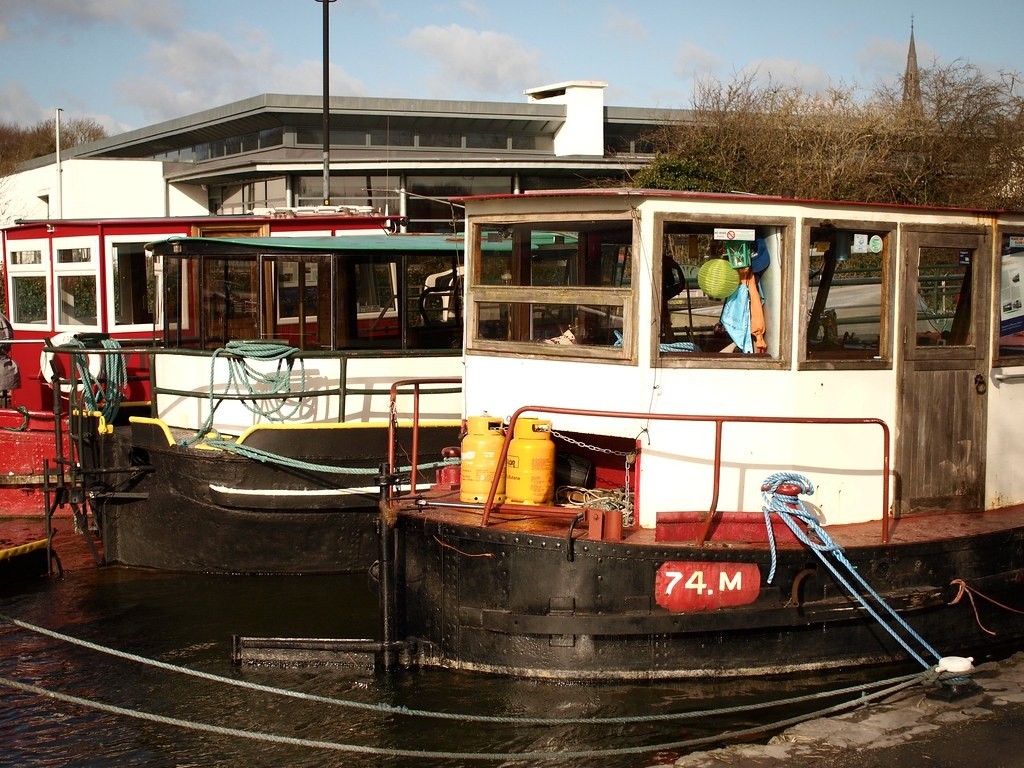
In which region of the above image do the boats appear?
[0,205,405,516]
[36,230,623,579]
[230,186,1024,708]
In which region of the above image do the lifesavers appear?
[40,332,102,392]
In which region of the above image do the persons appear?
[660,253,686,345]
[540,307,603,348]
